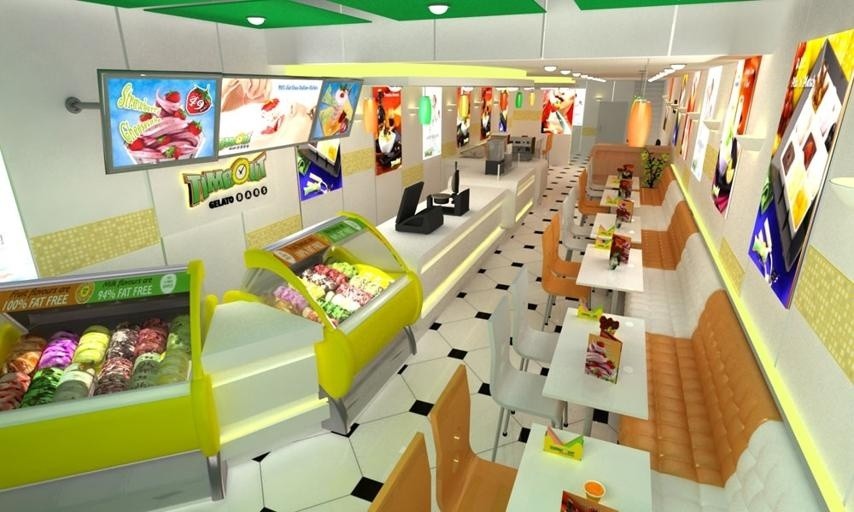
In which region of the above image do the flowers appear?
[640,149,672,187]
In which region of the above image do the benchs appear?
[617,164,828,512]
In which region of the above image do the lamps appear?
[626,58,654,148]
[545,67,608,85]
[247,16,265,25]
[647,64,687,84]
[428,4,447,15]
[418,85,537,125]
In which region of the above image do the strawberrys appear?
[187,84,211,113]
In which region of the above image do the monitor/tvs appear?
[395,181,424,224]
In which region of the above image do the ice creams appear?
[122,90,205,165]
[586,339,615,380]
